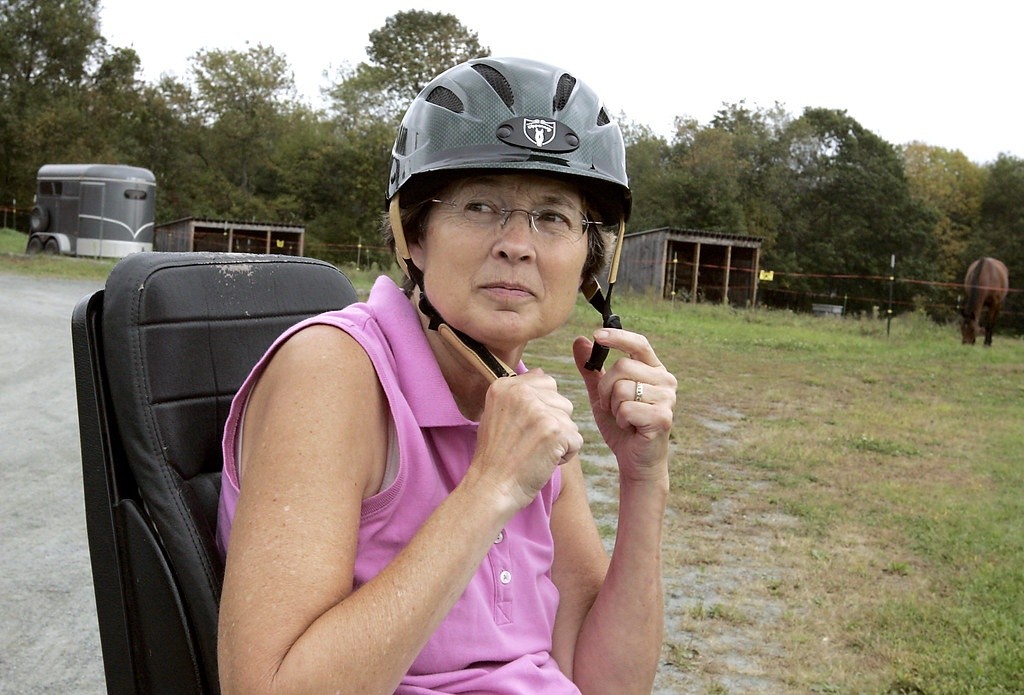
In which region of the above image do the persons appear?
[220,57,676,695]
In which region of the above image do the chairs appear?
[73,252,361,695]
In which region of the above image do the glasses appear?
[422,197,607,242]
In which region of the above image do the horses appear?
[957,256,1008,346]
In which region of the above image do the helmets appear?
[387,56,632,228]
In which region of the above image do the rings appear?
[636,381,643,400]
